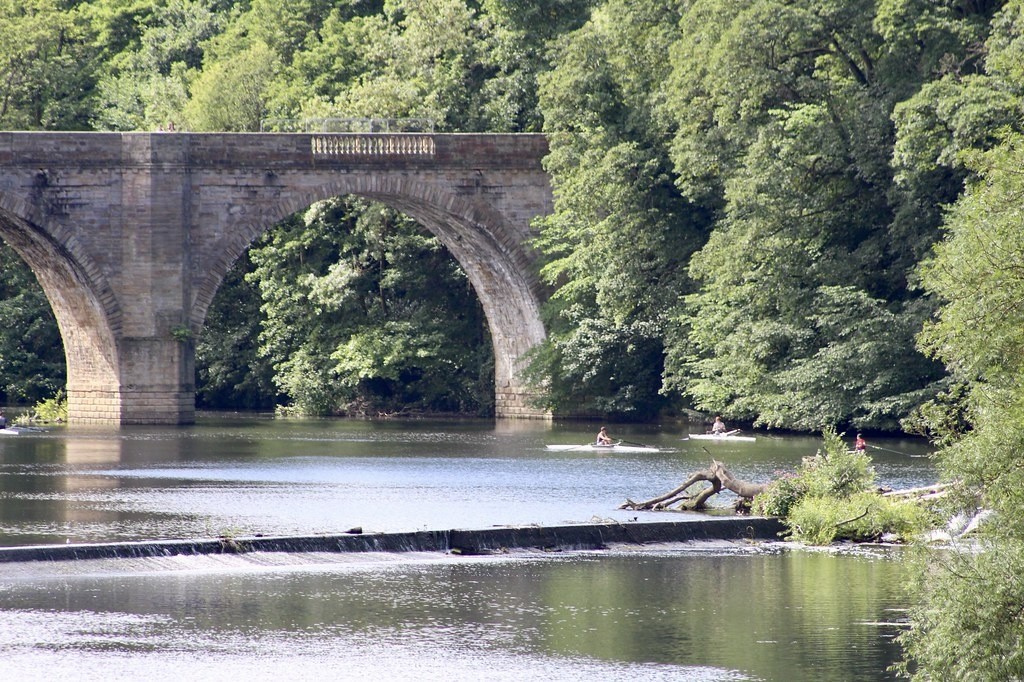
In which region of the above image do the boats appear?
[545,444,660,453]
[688,434,757,441]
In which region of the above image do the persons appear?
[855,433,866,450]
[597,427,612,445]
[706,417,726,434]
[0,410,7,429]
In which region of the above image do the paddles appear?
[861,443,913,457]
[608,436,655,448]
[691,428,739,435]
